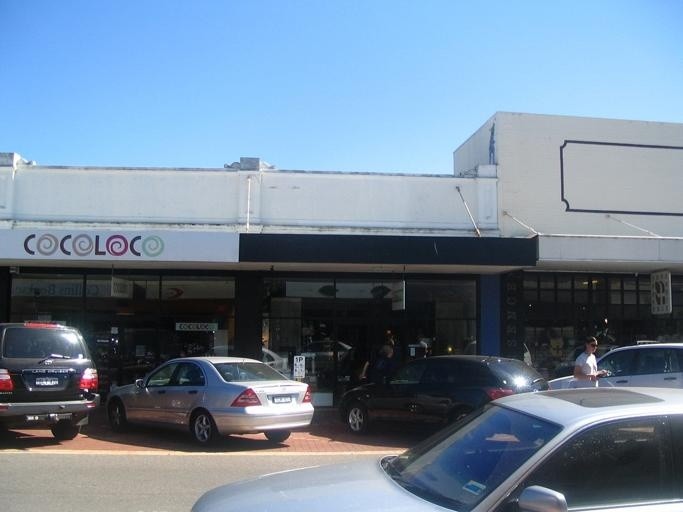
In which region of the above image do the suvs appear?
[0,322,102,441]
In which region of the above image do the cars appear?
[543,341,683,389]
[300,340,354,365]
[187,385,683,510]
[92,338,164,393]
[106,355,315,444]
[334,355,549,432]
[553,345,618,376]
[466,339,477,354]
[204,343,291,376]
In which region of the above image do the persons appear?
[572,335,607,387]
